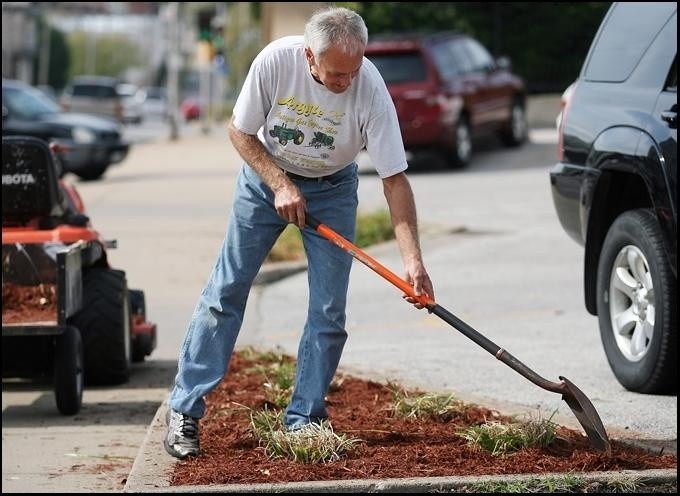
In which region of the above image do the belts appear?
[282,169,296,179]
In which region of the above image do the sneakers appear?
[164,408,200,460]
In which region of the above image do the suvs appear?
[1,76,135,187]
[543,1,678,398]
[362,28,534,174]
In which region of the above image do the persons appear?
[162,5,434,459]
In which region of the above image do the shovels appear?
[295,211,612,460]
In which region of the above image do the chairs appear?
[0,136,63,231]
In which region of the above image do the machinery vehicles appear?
[0,133,162,419]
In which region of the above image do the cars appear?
[38,69,204,127]
